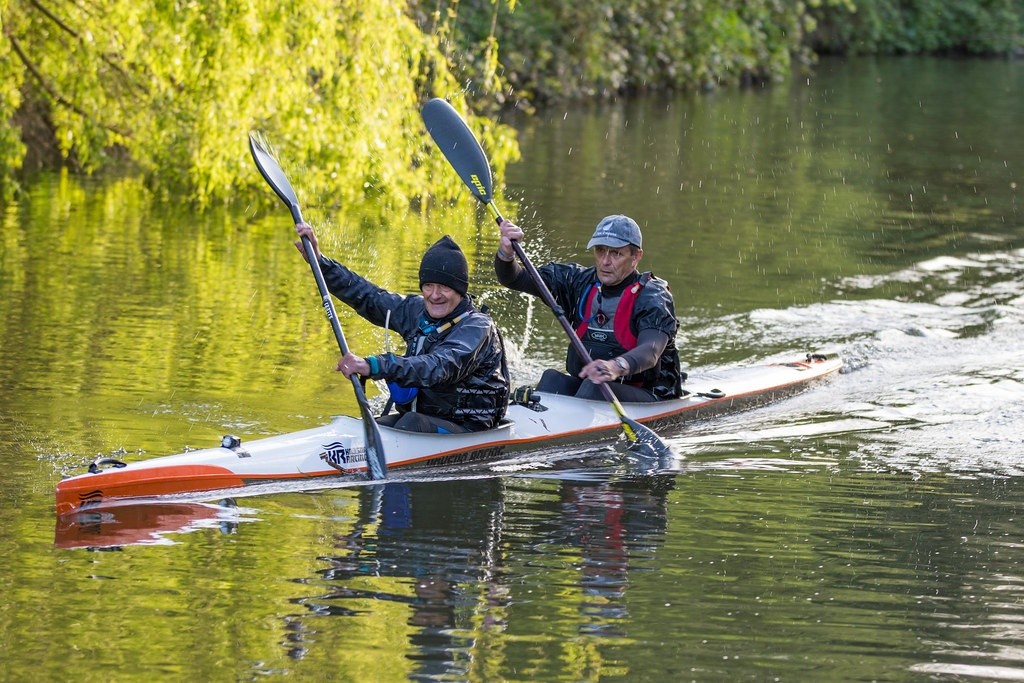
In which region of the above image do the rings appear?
[344,364,348,368]
[595,365,601,371]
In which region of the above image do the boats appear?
[50,343,850,515]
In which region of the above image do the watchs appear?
[610,357,626,376]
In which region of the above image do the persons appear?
[494,214,683,401]
[295,220,508,435]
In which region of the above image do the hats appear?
[586,214,642,250]
[419,234,468,297]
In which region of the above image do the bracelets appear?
[497,247,515,259]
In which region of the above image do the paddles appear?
[249,129,388,481]
[421,97,673,471]
